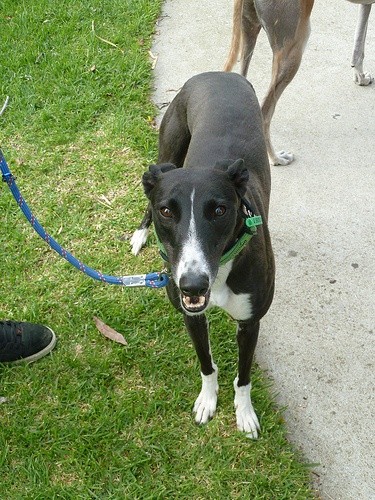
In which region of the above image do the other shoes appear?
[0,318,59,368]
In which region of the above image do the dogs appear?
[223,0,373,166]
[129,71,276,440]
